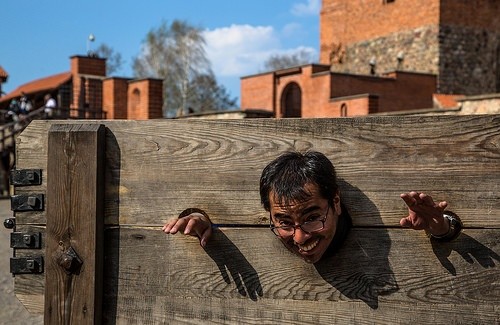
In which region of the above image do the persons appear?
[9,92,55,129]
[162,148,461,264]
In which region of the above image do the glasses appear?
[269,202,330,237]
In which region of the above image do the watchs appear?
[424,211,459,240]
